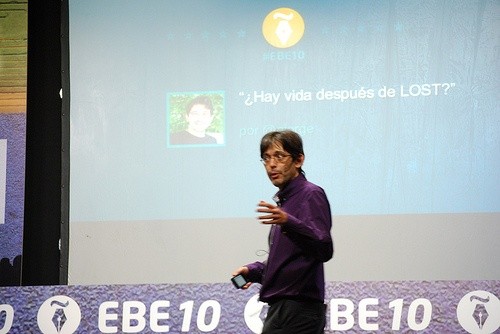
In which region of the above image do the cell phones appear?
[231,273,257,289]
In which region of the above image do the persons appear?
[167,96,219,145]
[233,130,335,334]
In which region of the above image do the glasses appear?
[260,151,293,166]
